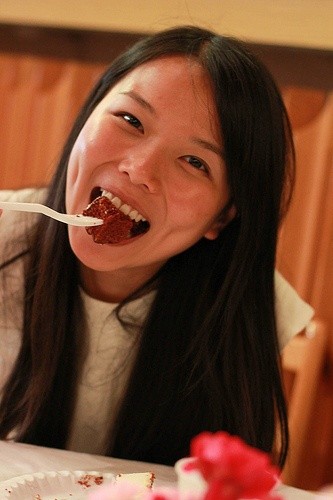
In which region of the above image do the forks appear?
[0,201,103,226]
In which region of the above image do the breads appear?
[83,197,136,245]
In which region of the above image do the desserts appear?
[174,431,284,500]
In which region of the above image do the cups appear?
[175,458,279,500]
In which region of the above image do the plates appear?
[0,472,117,500]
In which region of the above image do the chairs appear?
[272,317,327,492]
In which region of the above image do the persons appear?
[0,26,313,480]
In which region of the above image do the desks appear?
[0,441,332,500]
